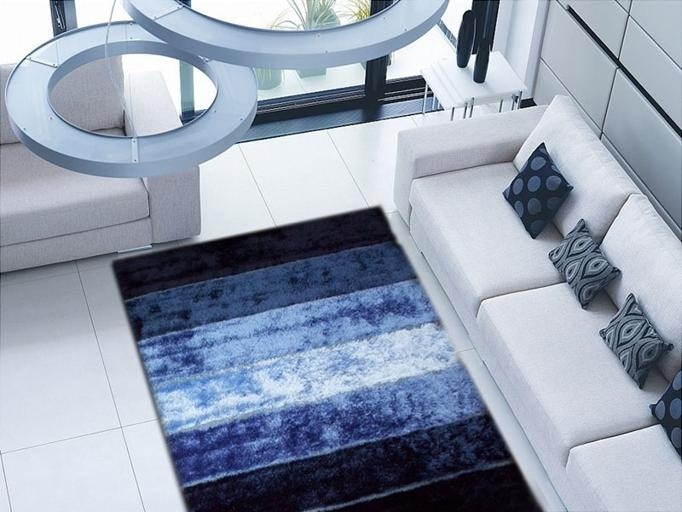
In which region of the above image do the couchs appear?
[385,91,682,511]
[1,53,203,277]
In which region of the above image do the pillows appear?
[648,368,682,462]
[547,217,623,311]
[597,291,676,391]
[500,140,574,240]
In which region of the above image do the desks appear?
[414,47,529,122]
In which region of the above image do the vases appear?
[455,9,476,69]
[472,40,491,85]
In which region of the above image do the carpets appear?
[109,202,542,512]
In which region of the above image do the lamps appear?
[3,17,260,181]
[118,0,451,72]
